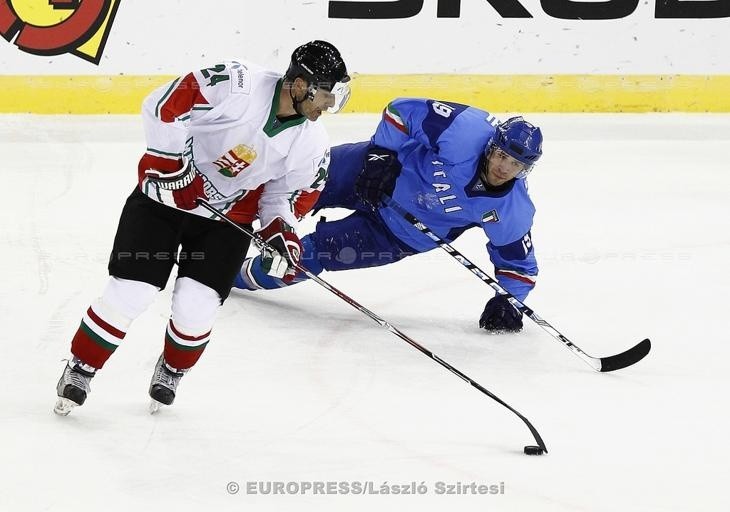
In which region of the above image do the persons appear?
[232,97,543,332]
[57,39,351,404]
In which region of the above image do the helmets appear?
[285,39,352,115]
[484,115,544,181]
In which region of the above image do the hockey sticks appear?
[380,189,651,372]
[194,197,549,457]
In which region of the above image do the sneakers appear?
[148,351,184,406]
[56,355,98,408]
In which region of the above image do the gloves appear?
[252,215,304,285]
[144,155,210,211]
[478,295,524,334]
[353,146,403,215]
